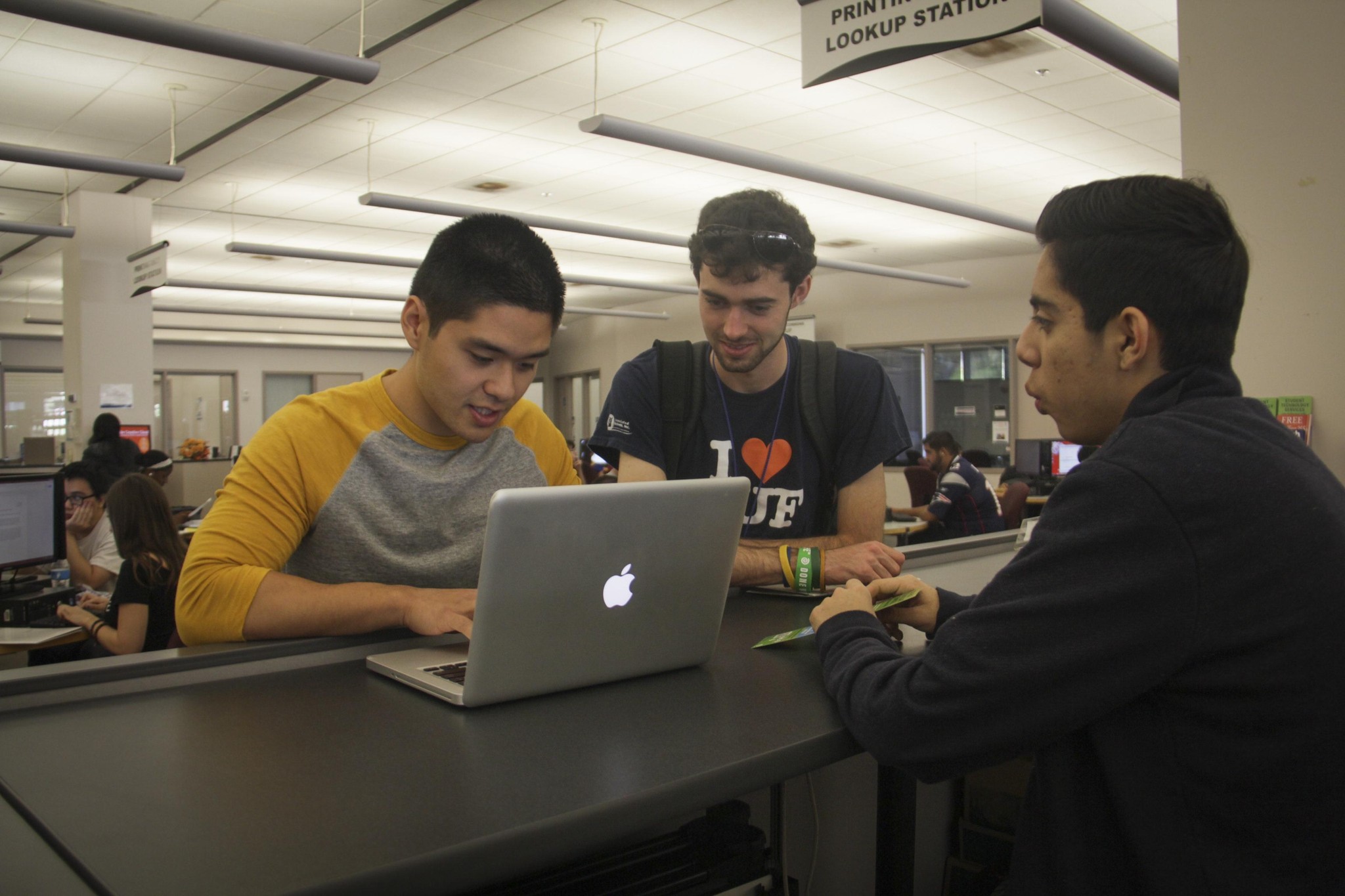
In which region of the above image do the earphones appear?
[941,452,945,456]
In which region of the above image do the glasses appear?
[699,224,810,264]
[65,493,101,506]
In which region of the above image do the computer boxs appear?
[0,586,76,628]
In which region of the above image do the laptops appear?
[364,474,752,708]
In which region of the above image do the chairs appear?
[999,481,1030,528]
[904,466,940,509]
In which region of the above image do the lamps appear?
[0,0,1180,341]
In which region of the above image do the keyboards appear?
[891,513,917,522]
[27,611,102,628]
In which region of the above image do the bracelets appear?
[90,619,105,639]
[778,544,825,595]
[94,622,108,641]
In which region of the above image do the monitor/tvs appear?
[1049,439,1086,479]
[119,424,152,456]
[579,438,609,471]
[23,436,55,466]
[1014,438,1051,478]
[0,473,67,570]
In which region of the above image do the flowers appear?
[178,436,211,460]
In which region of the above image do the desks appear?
[0,624,91,671]
[881,520,927,546]
[997,492,1052,506]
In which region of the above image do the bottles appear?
[50,559,71,606]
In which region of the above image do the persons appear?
[58,472,189,659]
[586,187,912,595]
[175,213,581,640]
[81,412,140,494]
[812,176,1345,896]
[566,439,608,485]
[135,449,191,526]
[891,430,1005,544]
[27,462,125,668]
